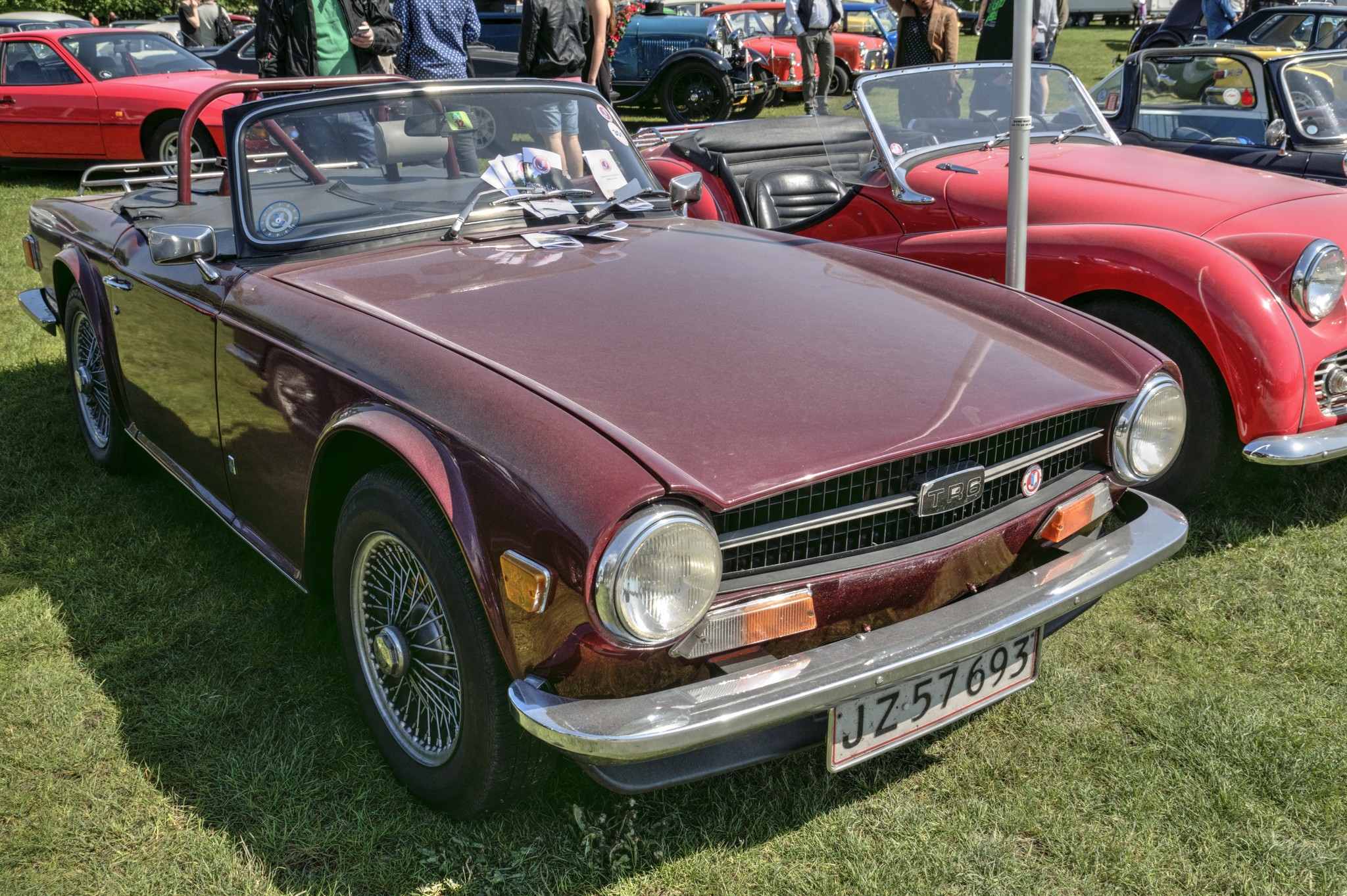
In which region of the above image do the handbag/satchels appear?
[945,74,964,101]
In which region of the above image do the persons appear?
[178,0,233,49]
[108,11,122,24]
[784,0,845,115]
[1200,0,1245,43]
[254,0,404,169]
[968,0,1069,120]
[88,12,100,27]
[392,0,481,176]
[885,0,959,130]
[516,1,617,180]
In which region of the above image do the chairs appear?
[91,56,121,81]
[15,60,46,85]
[745,165,850,228]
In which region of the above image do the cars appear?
[465,1,992,126]
[1065,0,1347,192]
[622,53,1347,520]
[14,70,1194,828]
[0,11,261,76]
[0,24,305,186]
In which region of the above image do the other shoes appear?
[806,111,819,116]
[817,111,834,116]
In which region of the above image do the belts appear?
[808,28,826,31]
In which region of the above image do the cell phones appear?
[354,25,369,37]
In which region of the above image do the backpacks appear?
[214,5,237,46]
